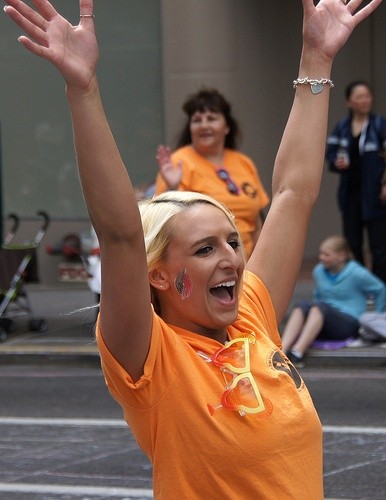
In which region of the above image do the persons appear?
[326,80,386,307]
[280,235,386,368]
[155,86,270,261]
[4,0,383,500]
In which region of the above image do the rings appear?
[79,13,97,19]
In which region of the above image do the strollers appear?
[0,207,53,344]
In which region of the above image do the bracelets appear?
[292,75,336,94]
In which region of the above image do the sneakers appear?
[285,350,305,368]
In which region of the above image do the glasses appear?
[211,336,266,414]
[216,168,240,196]
